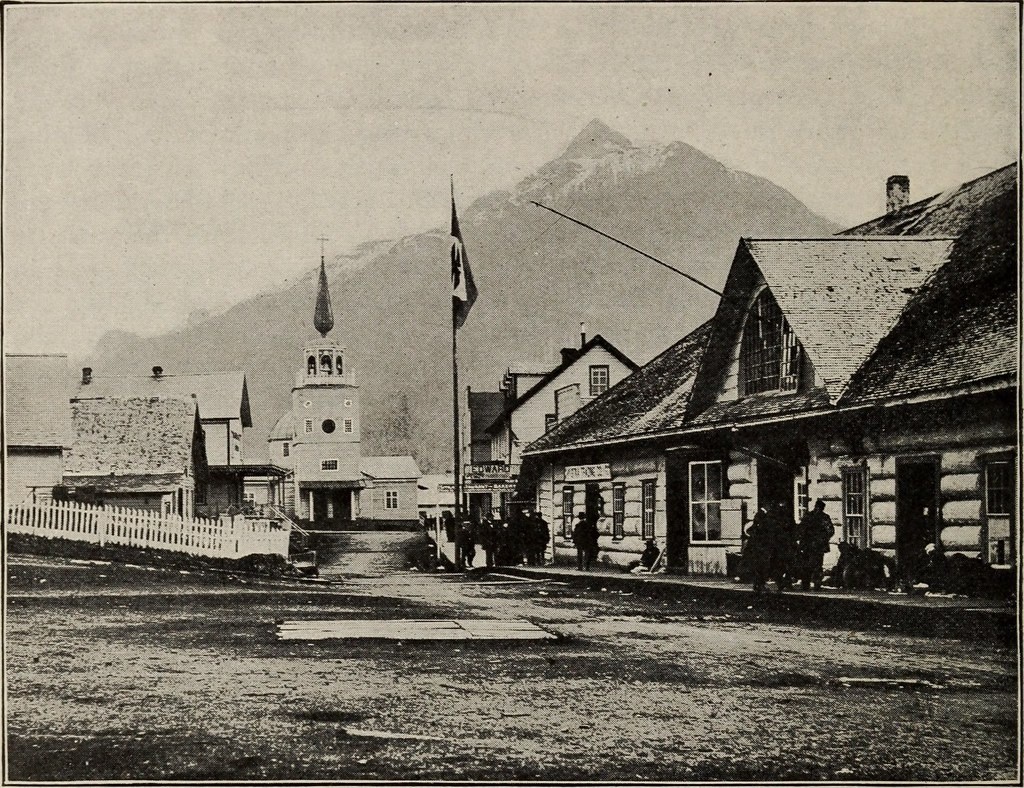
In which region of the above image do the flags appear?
[449,174,479,332]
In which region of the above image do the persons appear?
[420,505,598,573]
[734,494,1004,607]
[626,538,661,576]
